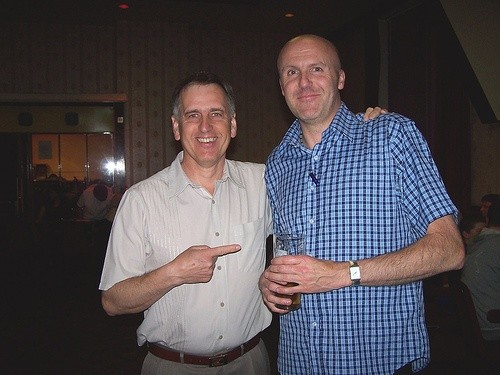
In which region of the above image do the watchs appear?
[345,260,361,287]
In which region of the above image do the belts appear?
[147,334,260,367]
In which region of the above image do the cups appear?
[273,232,303,310]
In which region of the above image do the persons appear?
[444,194,500,358]
[97,71,390,375]
[263,35,466,375]
[76,183,112,221]
